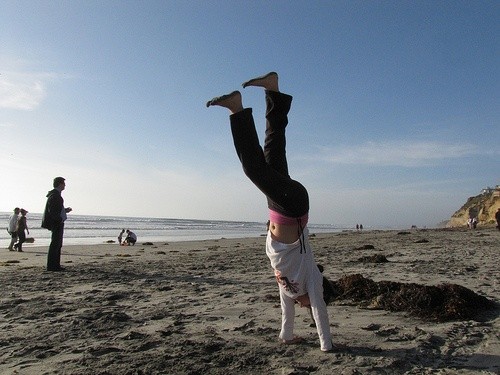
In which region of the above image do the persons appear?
[8,206,30,252]
[42,177,71,271]
[206,69,341,352]
[467,216,477,231]
[117,229,137,245]
[356,223,363,231]
[495,207,500,232]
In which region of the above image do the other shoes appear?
[48,267,67,272]
